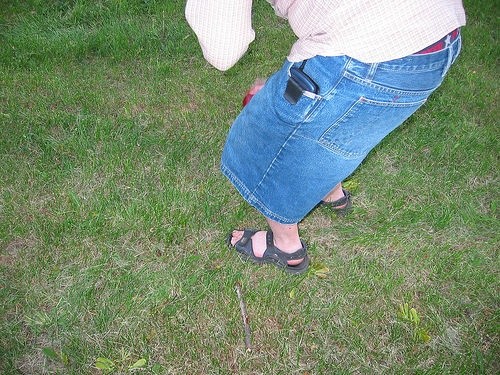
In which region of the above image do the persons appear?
[184,0,467,274]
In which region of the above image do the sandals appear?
[226,229,309,275]
[320,189,354,217]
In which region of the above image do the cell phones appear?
[290,66,320,94]
